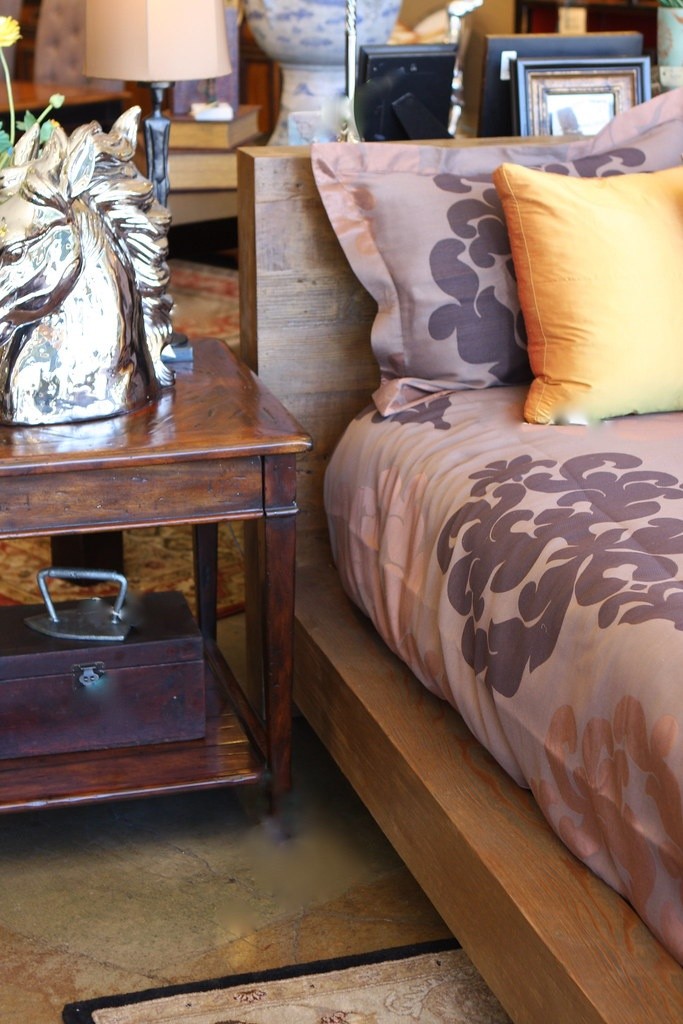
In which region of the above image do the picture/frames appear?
[508,55,651,142]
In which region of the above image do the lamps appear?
[85,0,234,359]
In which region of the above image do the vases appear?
[0,105,178,428]
[658,8,683,87]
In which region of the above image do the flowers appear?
[0,15,66,168]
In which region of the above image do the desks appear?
[0,80,132,138]
[0,338,317,841]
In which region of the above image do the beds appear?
[236,136,683,1023]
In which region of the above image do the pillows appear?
[310,87,683,415]
[491,163,683,425]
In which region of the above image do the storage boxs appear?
[0,565,208,761]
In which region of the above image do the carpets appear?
[2,256,245,625]
[62,940,511,1023]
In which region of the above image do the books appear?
[167,103,263,191]
